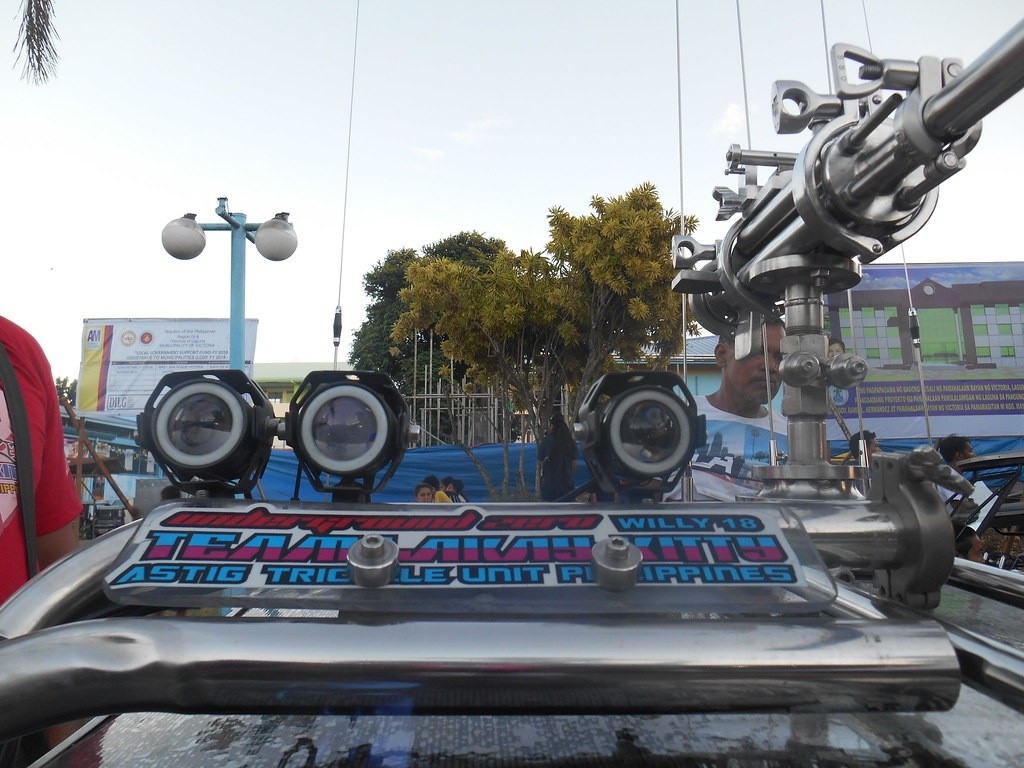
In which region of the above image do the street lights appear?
[161,194,300,369]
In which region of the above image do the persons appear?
[537,414,577,502]
[848,430,881,463]
[588,479,616,502]
[0,314,85,754]
[933,433,977,515]
[663,316,787,503]
[952,526,985,563]
[826,339,845,358]
[415,474,468,503]
